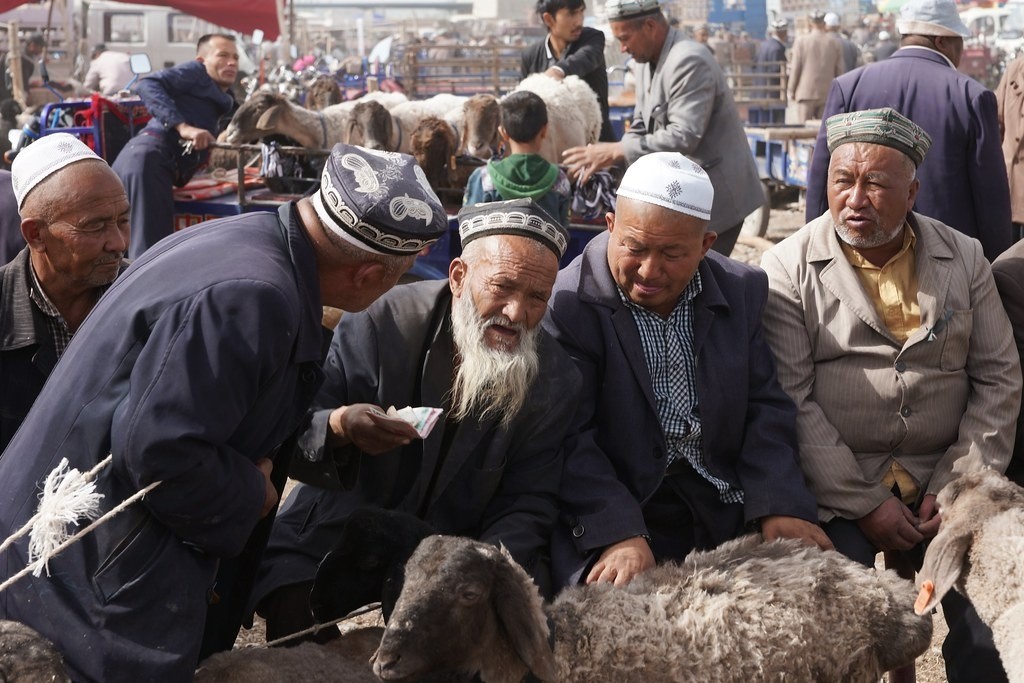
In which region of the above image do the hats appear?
[824,108,933,171]
[457,197,571,263]
[11,133,109,215]
[824,12,839,26]
[770,18,788,30]
[320,143,449,256]
[616,151,714,220]
[605,0,660,21]
[898,0,972,37]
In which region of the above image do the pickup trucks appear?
[0,0,264,78]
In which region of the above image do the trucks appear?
[607,59,822,241]
[3,33,615,284]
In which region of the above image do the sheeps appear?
[460,71,605,164]
[913,466,1024,683]
[0,618,391,683]
[345,91,473,156]
[225,91,411,177]
[371,534,933,683]
[412,107,465,183]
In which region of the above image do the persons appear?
[0,0,1024,683]
[538,152,836,599]
